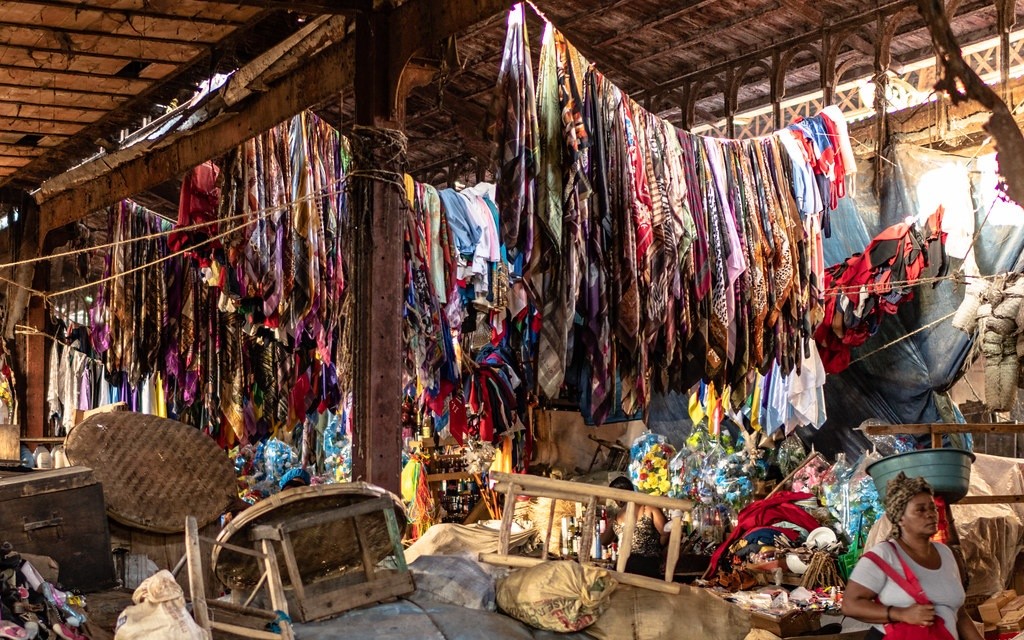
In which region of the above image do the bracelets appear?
[887,606,896,623]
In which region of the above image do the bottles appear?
[32,444,52,469]
[50,444,60,468]
[20,443,35,468]
[688,502,724,544]
[567,504,618,568]
[423,445,479,523]
[422,415,432,438]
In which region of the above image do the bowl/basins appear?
[865,449,976,502]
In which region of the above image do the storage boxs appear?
[247,493,418,624]
[0,463,119,597]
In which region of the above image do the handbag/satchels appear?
[857,540,956,640]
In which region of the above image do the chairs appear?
[169,516,296,640]
[478,472,694,596]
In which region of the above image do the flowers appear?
[323,416,353,484]
[630,426,885,543]
[235,438,299,480]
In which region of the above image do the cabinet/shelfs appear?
[407,435,496,525]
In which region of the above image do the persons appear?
[842,472,984,640]
[599,476,670,579]
